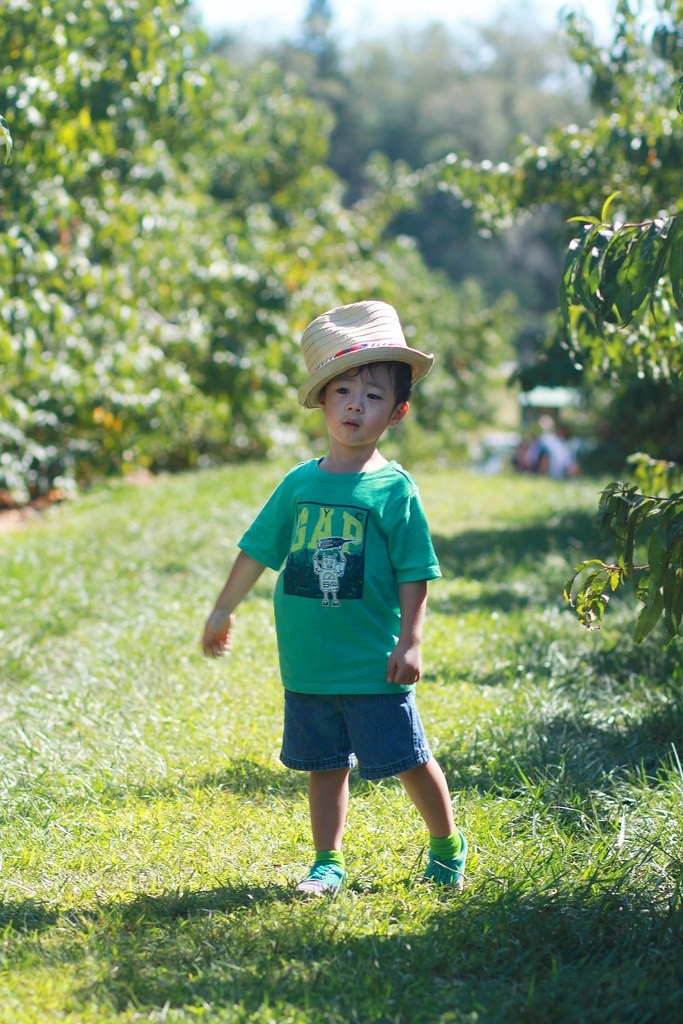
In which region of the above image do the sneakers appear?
[297,862,347,897]
[418,832,468,895]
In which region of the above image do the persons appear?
[202,356,467,897]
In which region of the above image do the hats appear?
[298,300,434,409]
